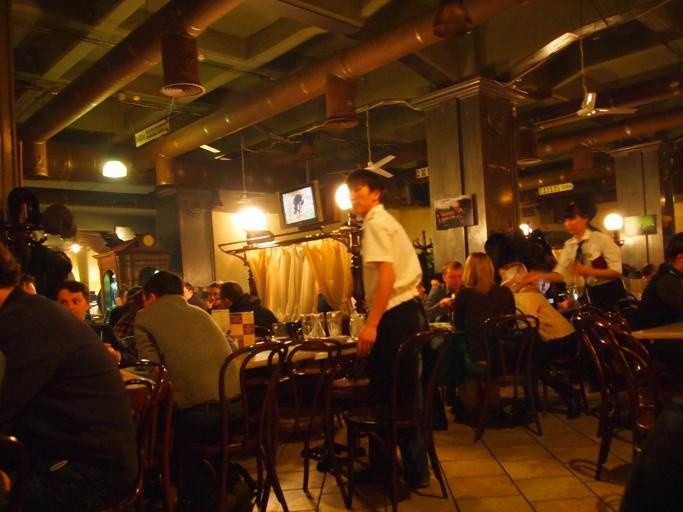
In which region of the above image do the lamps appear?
[224,133,275,233]
[333,183,358,231]
[98,141,130,181]
[602,212,627,248]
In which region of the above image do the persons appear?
[0,239,284,512]
[343,169,433,488]
[424,196,682,421]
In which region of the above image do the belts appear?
[208,397,241,406]
[384,298,419,313]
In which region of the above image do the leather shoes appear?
[432,425,448,431]
[353,468,397,490]
[400,465,431,488]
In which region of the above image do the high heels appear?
[567,389,586,418]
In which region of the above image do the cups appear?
[209,308,231,336]
[299,310,365,343]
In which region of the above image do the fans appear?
[535,26,637,132]
[357,100,397,177]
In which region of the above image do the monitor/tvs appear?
[274,178,324,229]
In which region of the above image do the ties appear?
[575,239,586,264]
